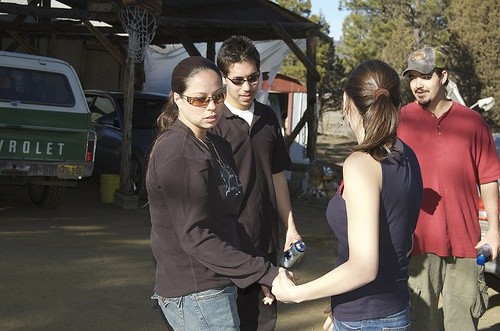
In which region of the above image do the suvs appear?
[0,51,97,208]
[84,89,173,201]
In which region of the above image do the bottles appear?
[476,244,493,266]
[280,240,306,269]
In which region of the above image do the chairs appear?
[139,101,162,129]
[296,164,336,203]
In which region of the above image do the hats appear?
[400,47,447,77]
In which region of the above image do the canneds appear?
[280,240,306,270]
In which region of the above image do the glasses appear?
[226,70,261,85]
[181,92,226,107]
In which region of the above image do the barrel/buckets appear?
[100,174,121,203]
